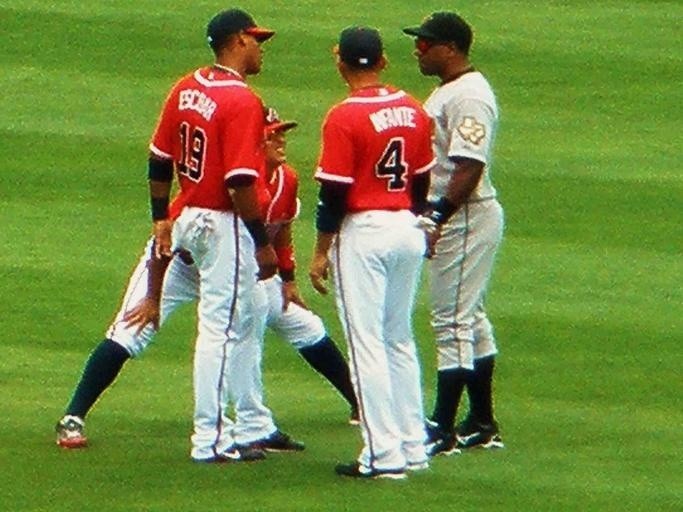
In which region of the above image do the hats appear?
[405,12,472,46]
[334,26,381,65]
[265,108,297,130]
[207,10,274,44]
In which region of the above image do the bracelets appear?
[241,216,271,250]
[146,195,171,225]
[428,194,455,226]
[278,268,296,284]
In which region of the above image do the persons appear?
[54,103,365,448]
[399,12,509,458]
[147,7,307,464]
[309,25,436,482]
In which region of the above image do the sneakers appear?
[260,430,306,453]
[422,415,462,457]
[218,443,265,461]
[57,420,86,447]
[455,422,504,449]
[348,410,362,425]
[336,462,407,480]
